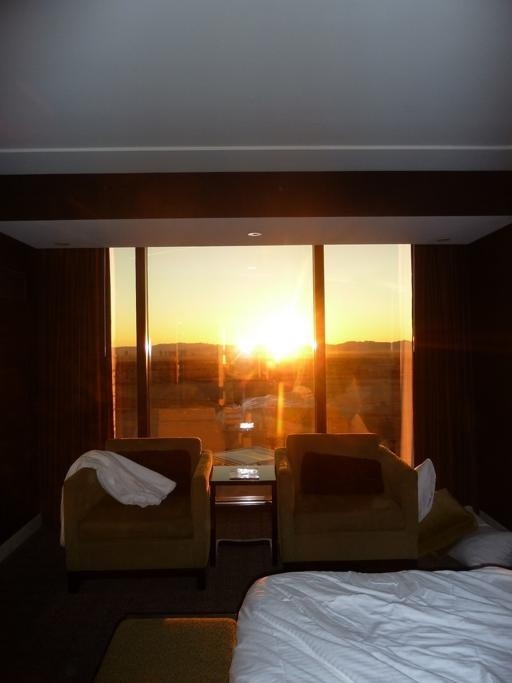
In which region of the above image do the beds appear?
[235,570,512,681]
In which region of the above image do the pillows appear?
[451,530,511,569]
[300,451,383,498]
[420,486,477,566]
[116,450,189,506]
[414,458,436,522]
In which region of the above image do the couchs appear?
[65,437,213,584]
[275,432,423,569]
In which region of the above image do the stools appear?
[100,615,235,681]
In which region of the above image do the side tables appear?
[211,464,276,561]
